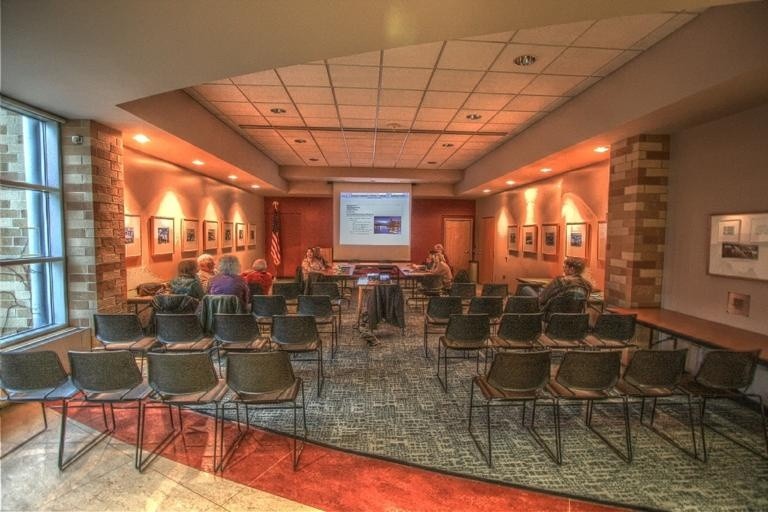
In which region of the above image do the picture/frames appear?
[748,217,767,245]
[507,224,520,253]
[181,217,199,252]
[595,220,607,261]
[564,222,589,260]
[704,209,768,283]
[149,214,175,258]
[521,227,538,254]
[540,223,560,258]
[202,220,258,250]
[716,217,741,245]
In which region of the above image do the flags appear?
[269,200,282,267]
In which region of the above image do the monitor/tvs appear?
[379,272,391,285]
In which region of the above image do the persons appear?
[519,257,591,321]
[169,259,205,300]
[196,252,220,293]
[207,255,250,311]
[301,248,340,304]
[424,242,454,291]
[241,258,275,296]
[313,246,333,269]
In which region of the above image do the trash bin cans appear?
[468,260,479,283]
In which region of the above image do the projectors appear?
[368,273,379,280]
